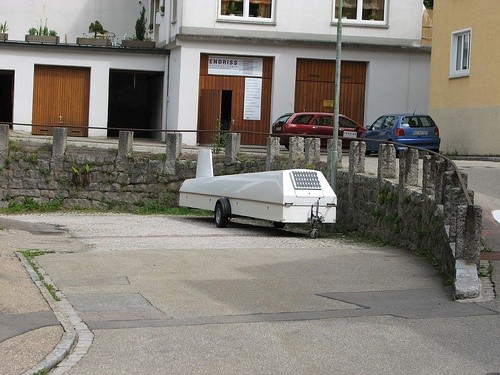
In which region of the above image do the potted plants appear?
[23,17,60,45]
[0,20,8,41]
[76,19,113,47]
[120,7,156,49]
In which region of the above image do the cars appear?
[272,112,368,151]
[360,106,440,158]
